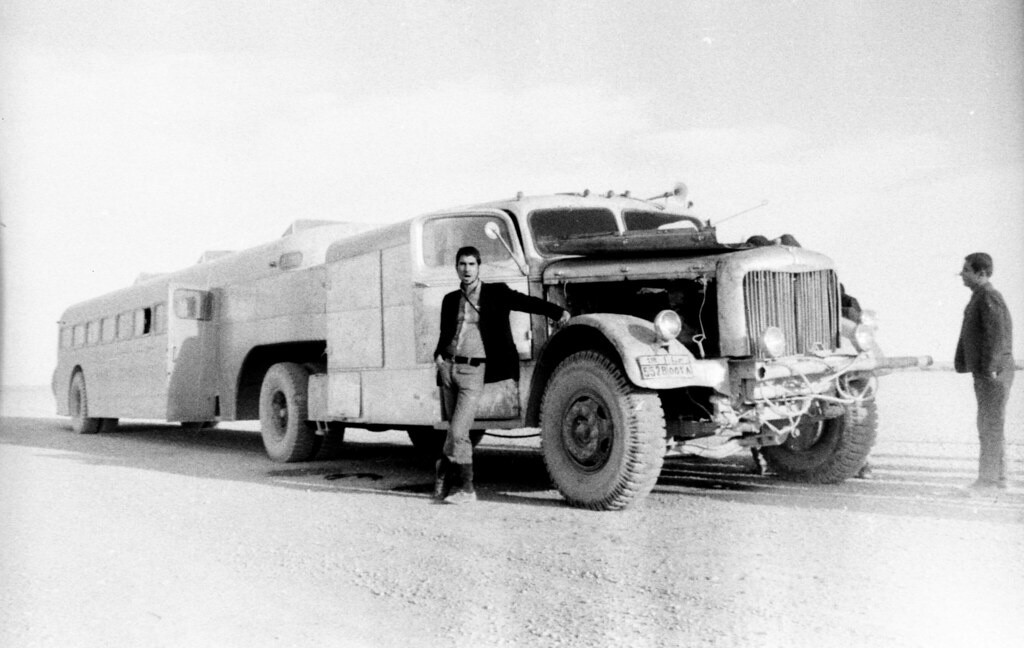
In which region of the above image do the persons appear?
[436,246,571,505]
[954,254,1014,494]
[839,284,876,481]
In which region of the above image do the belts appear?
[441,351,488,368]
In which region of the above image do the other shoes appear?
[444,491,478,503]
[857,466,874,480]
[433,459,448,501]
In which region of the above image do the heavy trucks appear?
[50,183,930,513]
